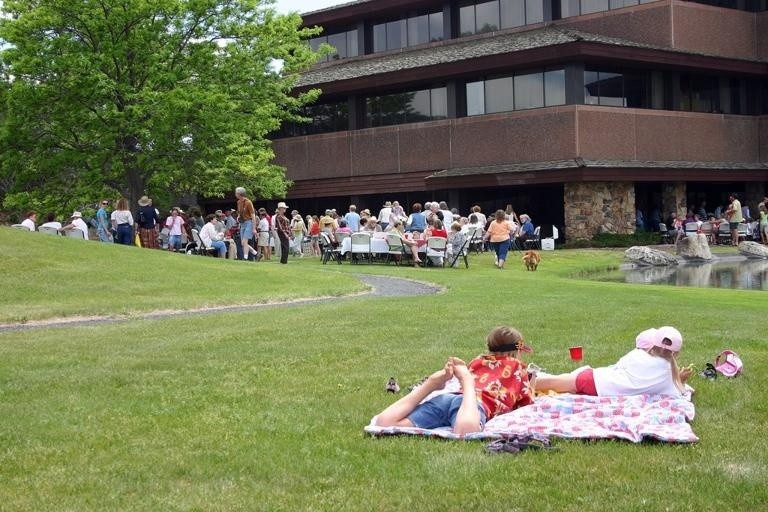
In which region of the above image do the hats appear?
[489,343,532,353]
[383,201,393,208]
[137,196,152,206]
[278,201,302,220]
[369,216,378,222]
[635,326,682,352]
[363,209,371,215]
[71,210,82,218]
[321,209,348,225]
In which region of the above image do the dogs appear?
[522,251,541,271]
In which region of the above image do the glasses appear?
[102,202,111,206]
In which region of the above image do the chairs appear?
[10,219,558,268]
[659,212,750,246]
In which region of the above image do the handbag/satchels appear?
[135,233,141,246]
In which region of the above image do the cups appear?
[569,346,583,362]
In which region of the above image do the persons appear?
[43,212,63,235]
[635,191,768,247]
[58,211,89,241]
[235,187,263,261]
[289,200,534,268]
[21,212,38,231]
[376,324,694,434]
[95,196,294,264]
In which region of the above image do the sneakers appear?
[255,252,263,262]
